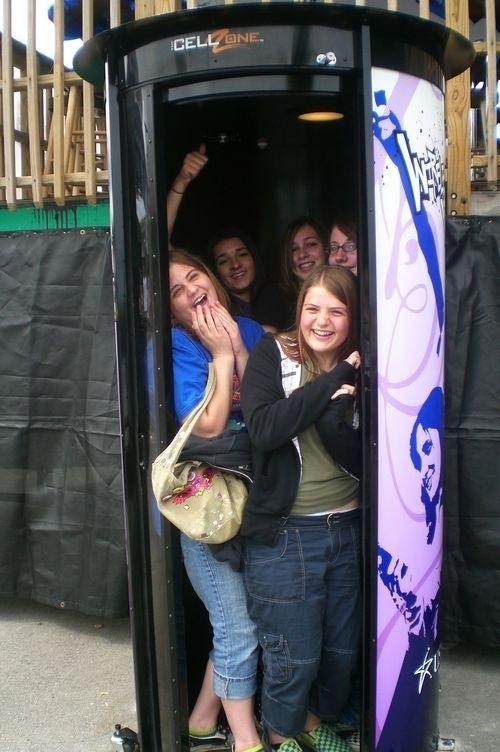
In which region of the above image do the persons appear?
[166,137,266,324]
[324,208,367,275]
[167,246,264,751]
[269,209,325,330]
[242,264,360,749]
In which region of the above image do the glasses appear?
[324,242,357,254]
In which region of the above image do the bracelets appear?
[170,184,188,197]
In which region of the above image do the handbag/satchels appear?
[150,359,249,545]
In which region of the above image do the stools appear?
[41,94,108,197]
[458,53,500,190]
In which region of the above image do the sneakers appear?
[183,725,233,751]
[261,714,361,752]
